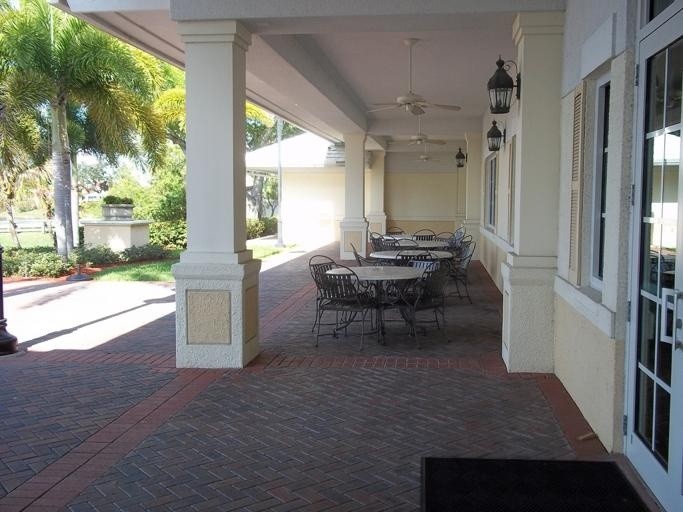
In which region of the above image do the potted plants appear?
[100,196,135,221]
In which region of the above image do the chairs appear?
[309,226,476,351]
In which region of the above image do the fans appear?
[367,38,461,163]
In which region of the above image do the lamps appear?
[486,54,521,151]
[456,148,468,167]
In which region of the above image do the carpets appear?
[421,457,653,512]
